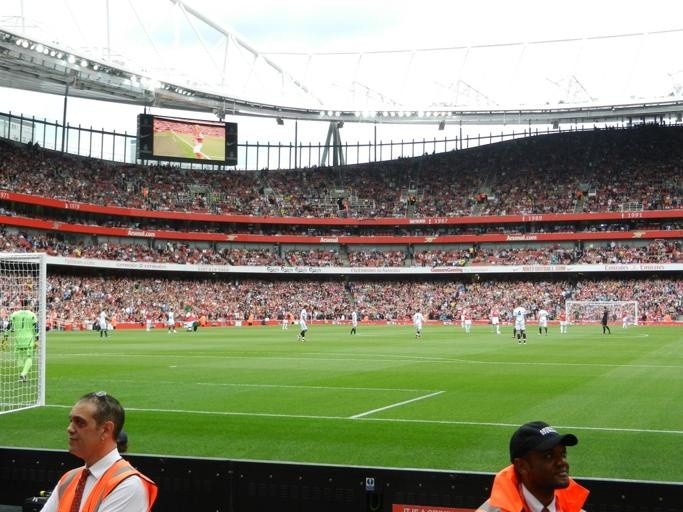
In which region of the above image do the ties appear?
[68,468,92,512]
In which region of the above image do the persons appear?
[511,328,522,338]
[37,390,159,512]
[512,301,527,344]
[411,308,425,339]
[6,296,39,383]
[471,419,591,512]
[598,305,610,336]
[296,304,308,342]
[99,307,112,338]
[1,121,683,334]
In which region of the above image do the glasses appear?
[94,389,114,417]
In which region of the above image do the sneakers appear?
[168,330,177,334]
[19,374,28,382]
[512,334,527,345]
[296,334,305,342]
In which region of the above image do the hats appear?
[509,419,579,463]
[117,430,127,443]
